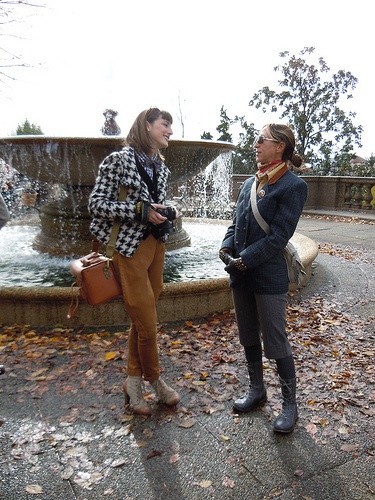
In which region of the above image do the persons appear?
[89,108,181,414]
[219,123,308,435]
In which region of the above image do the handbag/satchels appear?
[67,252,121,319]
[283,241,305,285]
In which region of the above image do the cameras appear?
[157,206,175,226]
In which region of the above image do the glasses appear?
[253,135,277,144]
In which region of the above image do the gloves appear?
[218,247,247,275]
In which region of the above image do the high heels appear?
[150,376,181,406]
[124,375,152,415]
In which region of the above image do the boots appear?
[233,360,267,412]
[273,374,298,433]
